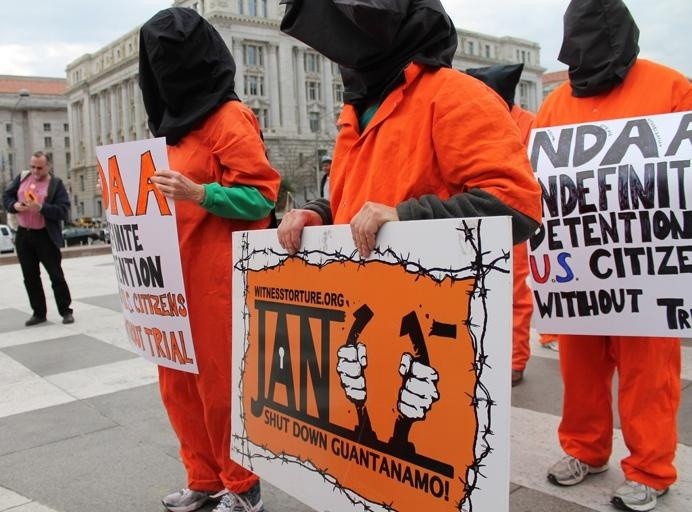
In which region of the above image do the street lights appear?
[315,103,344,198]
[10,89,29,148]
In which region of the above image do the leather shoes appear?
[24,315,46,326]
[63,313,74,324]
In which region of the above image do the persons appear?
[91,1,288,509]
[519,2,692,508]
[459,58,547,390]
[275,1,544,512]
[1,150,76,326]
[317,152,335,201]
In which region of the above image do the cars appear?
[0,217,111,253]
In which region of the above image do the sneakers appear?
[547,454,609,486]
[512,370,523,386]
[209,480,263,512]
[609,479,669,512]
[161,487,221,512]
[544,340,559,351]
